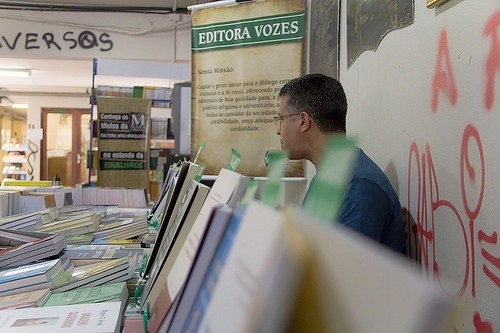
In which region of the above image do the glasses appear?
[272,113,315,124]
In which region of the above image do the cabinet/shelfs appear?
[2,148,27,181]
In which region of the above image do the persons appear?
[276,72,402,256]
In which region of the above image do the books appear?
[98,84,175,200]
[0,160,445,333]
[2,139,26,180]
